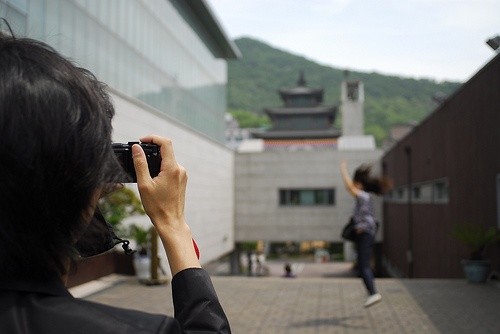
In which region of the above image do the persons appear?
[337,159,395,308]
[280,263,297,278]
[0,17,232,334]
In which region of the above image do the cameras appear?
[102,141,161,183]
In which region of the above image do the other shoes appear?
[364,293,381,307]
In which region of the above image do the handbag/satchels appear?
[342,218,358,242]
